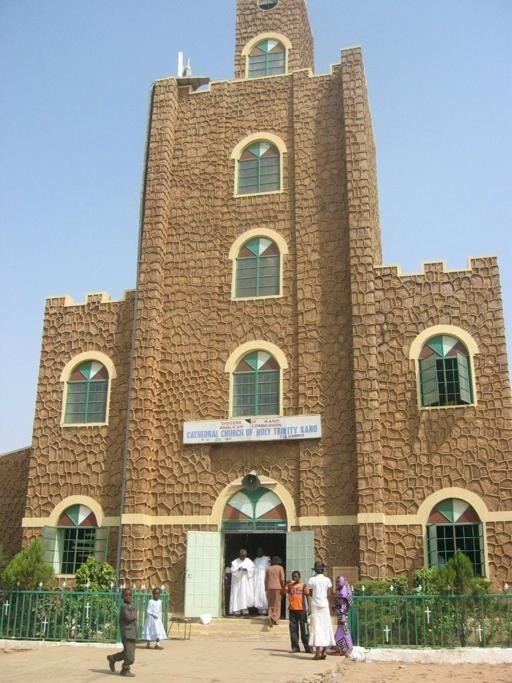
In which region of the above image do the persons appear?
[264,555,286,628]
[107,588,138,677]
[228,548,255,616]
[331,574,354,656]
[308,560,333,660]
[141,586,169,649]
[253,546,272,615]
[280,570,315,653]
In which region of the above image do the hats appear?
[268,555,283,564]
[311,561,325,570]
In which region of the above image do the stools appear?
[166,613,192,641]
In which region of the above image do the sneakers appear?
[290,646,348,659]
[107,655,115,671]
[120,668,136,676]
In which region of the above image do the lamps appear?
[242,469,264,492]
